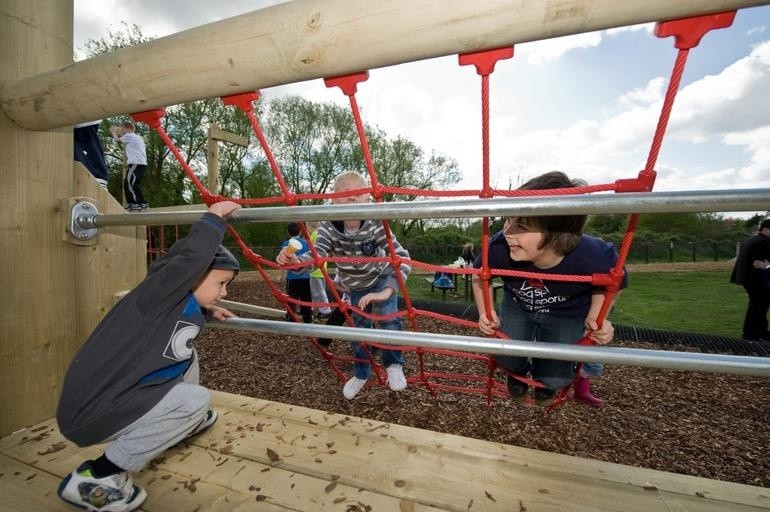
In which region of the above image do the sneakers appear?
[343,376,367,401]
[59,459,148,512]
[385,364,408,393]
[183,410,218,437]
[122,204,148,210]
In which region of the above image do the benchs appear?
[426,278,452,300]
[462,277,504,303]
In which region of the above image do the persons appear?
[73,118,109,188]
[728,217,770,341]
[573,358,605,407]
[279,172,411,399]
[110,123,153,213]
[471,172,626,400]
[462,242,477,263]
[318,295,380,354]
[54,198,242,512]
[305,220,332,322]
[752,259,770,271]
[280,223,314,323]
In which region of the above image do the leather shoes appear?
[506,372,528,398]
[533,385,555,407]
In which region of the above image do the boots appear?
[574,377,604,407]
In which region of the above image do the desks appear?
[443,266,475,302]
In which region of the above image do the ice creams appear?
[283,238,303,255]
